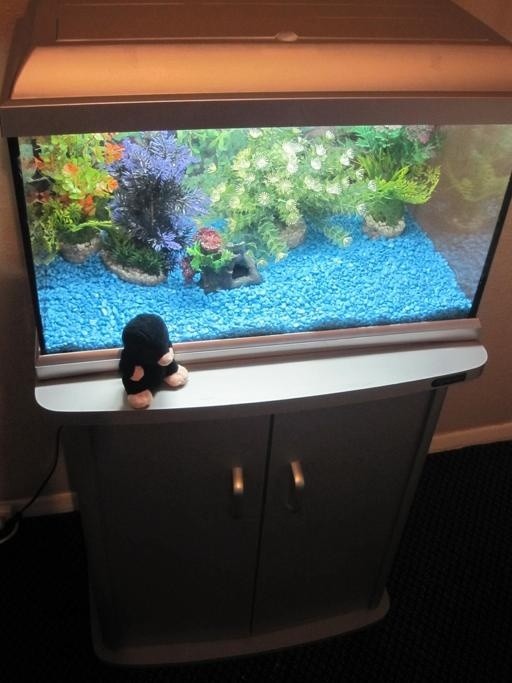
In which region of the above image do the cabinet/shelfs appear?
[64,386,450,665]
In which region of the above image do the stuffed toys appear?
[119,314,189,409]
